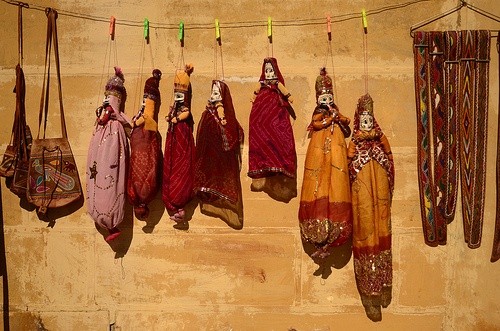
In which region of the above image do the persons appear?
[195,81,245,204]
[346,94,394,296]
[127,69,162,217]
[85,75,127,242]
[161,70,196,223]
[299,75,352,260]
[248,58,297,180]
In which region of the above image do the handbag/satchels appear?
[26,138,83,213]
[0,125,32,199]
